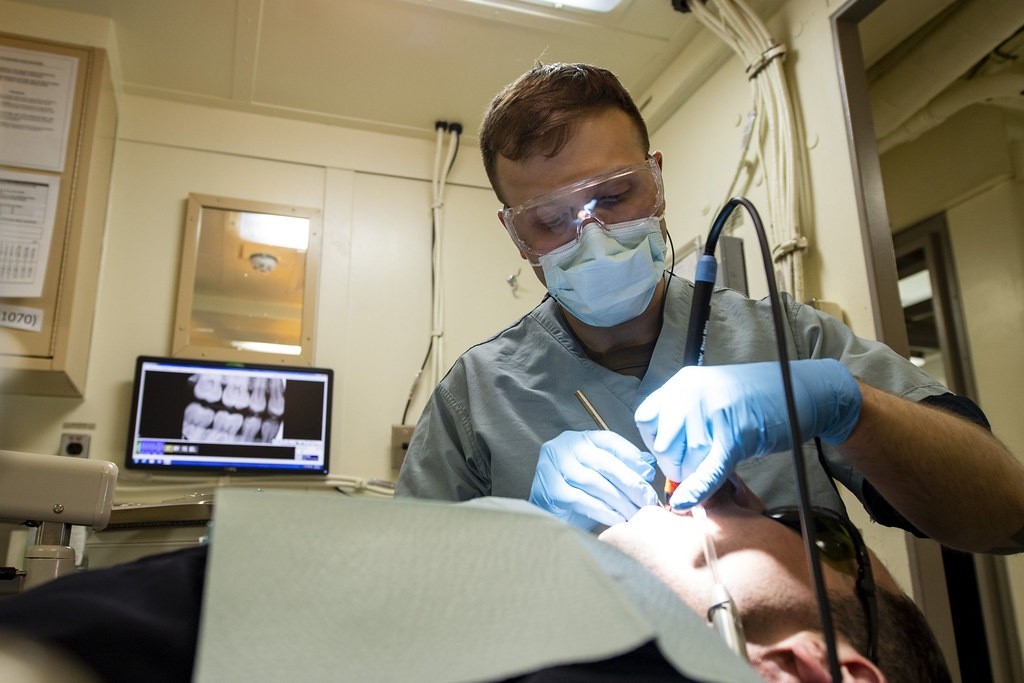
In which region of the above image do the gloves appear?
[635,355,864,512]
[528,428,661,532]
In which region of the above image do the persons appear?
[0,499,951,682]
[395,63,1024,555]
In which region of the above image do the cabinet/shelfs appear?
[0,37,121,400]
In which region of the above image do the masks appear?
[536,215,668,330]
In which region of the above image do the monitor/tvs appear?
[124,355,335,474]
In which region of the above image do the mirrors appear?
[172,191,322,368]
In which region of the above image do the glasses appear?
[767,501,884,665]
[497,152,668,264]
